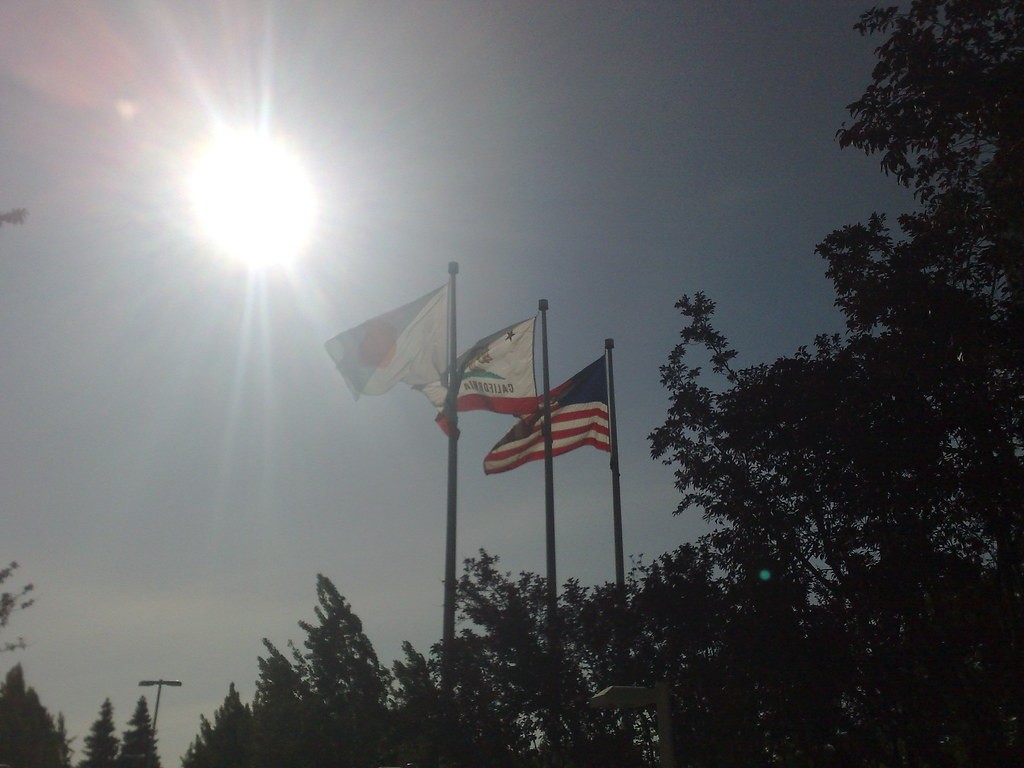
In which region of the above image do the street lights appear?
[138,676,182,744]
[590,679,677,768]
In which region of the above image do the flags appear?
[324,282,451,406]
[435,315,538,439]
[483,355,612,475]
